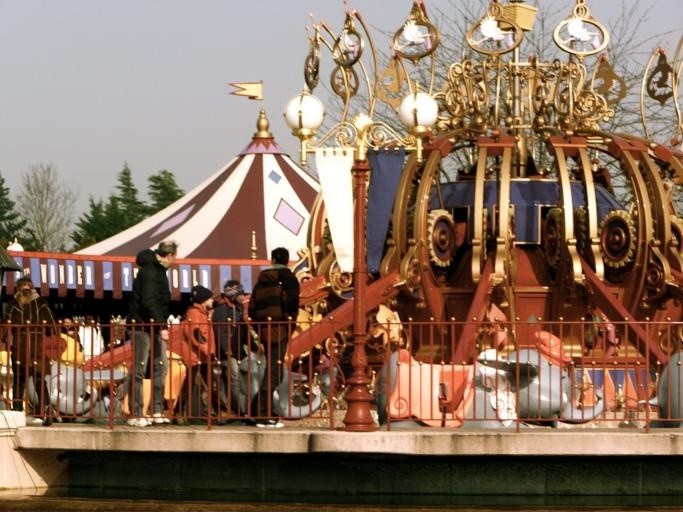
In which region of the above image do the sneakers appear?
[126,412,286,428]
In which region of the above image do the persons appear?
[170,282,239,425]
[75,314,105,363]
[62,314,80,342]
[124,239,178,428]
[3,273,60,426]
[209,278,259,427]
[246,245,301,431]
[164,307,186,327]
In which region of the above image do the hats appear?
[192,286,213,305]
[158,241,178,254]
